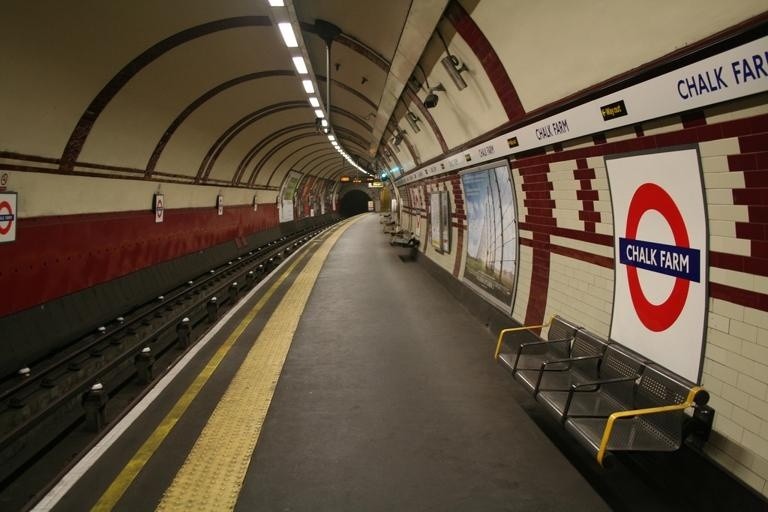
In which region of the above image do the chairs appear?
[495,316,714,474]
[380,211,418,249]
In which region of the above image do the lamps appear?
[268,0,469,185]
